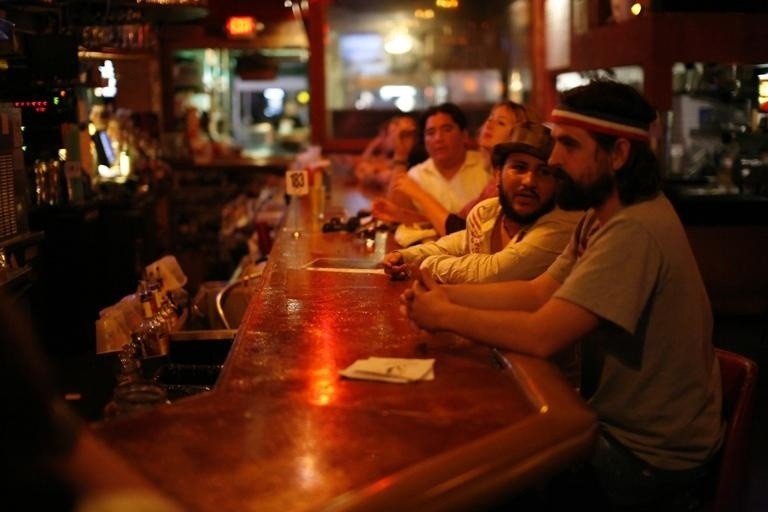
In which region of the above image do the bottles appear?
[91,253,189,384]
[99,382,172,419]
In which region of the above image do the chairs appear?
[712,345,759,512]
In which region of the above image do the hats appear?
[489,121,555,167]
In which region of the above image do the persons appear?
[395,78,727,510]
[378,118,586,394]
[174,105,214,165]
[333,98,539,247]
